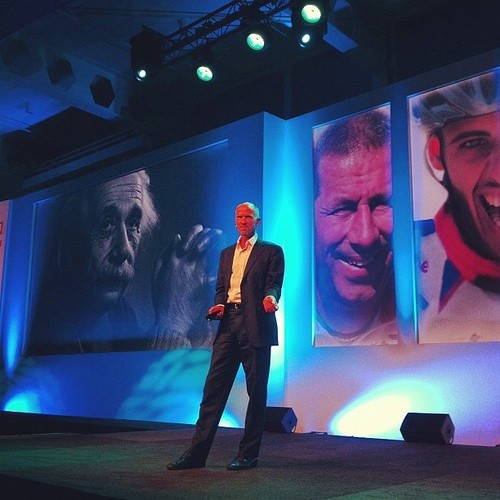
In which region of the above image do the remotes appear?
[206,310,221,321]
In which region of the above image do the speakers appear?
[264,407,297,434]
[399,413,456,445]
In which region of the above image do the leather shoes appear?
[167,456,206,471]
[226,457,258,469]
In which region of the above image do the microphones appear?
[235,225,238,228]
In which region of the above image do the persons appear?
[27,169,222,351]
[412,70,500,344]
[167,201,284,470]
[313,110,398,346]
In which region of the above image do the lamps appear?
[133,0,335,83]
[401,412,455,444]
[265,406,297,433]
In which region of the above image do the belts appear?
[225,303,243,309]
